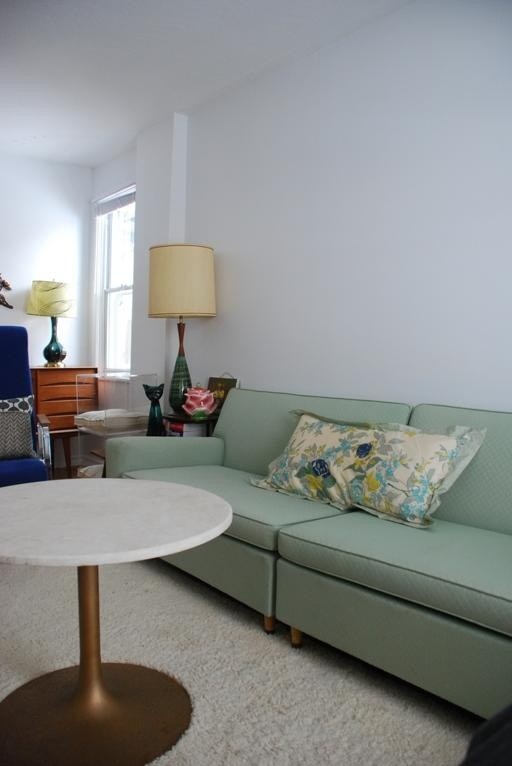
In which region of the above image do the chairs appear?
[1,326,53,488]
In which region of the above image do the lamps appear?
[25,279,78,367]
[149,244,217,413]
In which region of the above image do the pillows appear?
[0,393,38,458]
[247,411,487,529]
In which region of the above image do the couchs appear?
[103,388,512,722]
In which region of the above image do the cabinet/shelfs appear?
[73,372,159,476]
[31,368,99,478]
